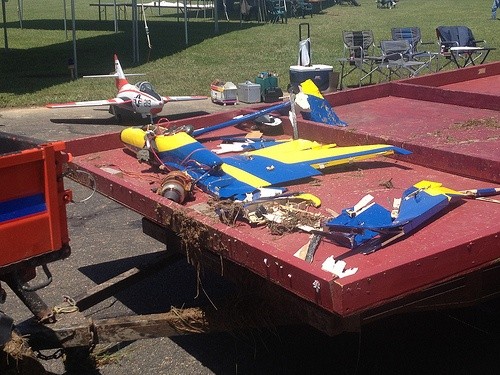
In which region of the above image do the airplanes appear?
[44,52,208,124]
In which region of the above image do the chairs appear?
[290,0,313,19]
[268,0,287,24]
[335,30,389,91]
[391,27,440,78]
[380,40,431,82]
[435,26,496,71]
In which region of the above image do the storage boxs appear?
[238,82,261,103]
[289,64,333,94]
[255,73,278,101]
[211,84,237,105]
